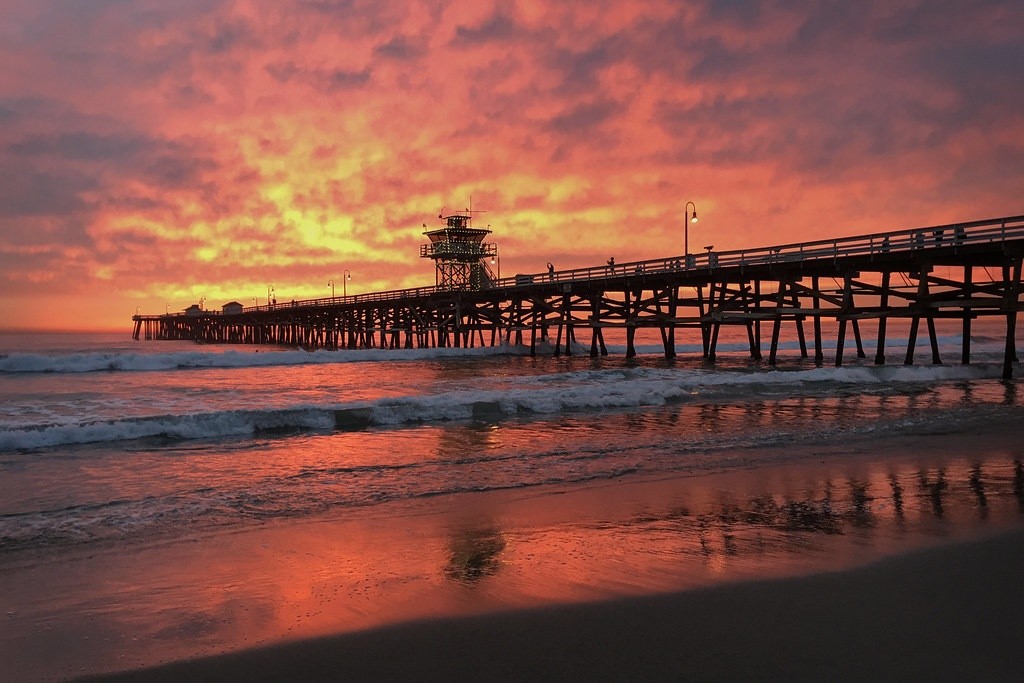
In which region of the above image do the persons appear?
[547,262,555,282]
[607,257,617,276]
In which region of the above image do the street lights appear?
[252,296,258,306]
[267,284,274,305]
[200,294,206,311]
[684,201,699,254]
[165,303,171,314]
[342,269,350,302]
[327,280,335,305]
[490,251,500,287]
